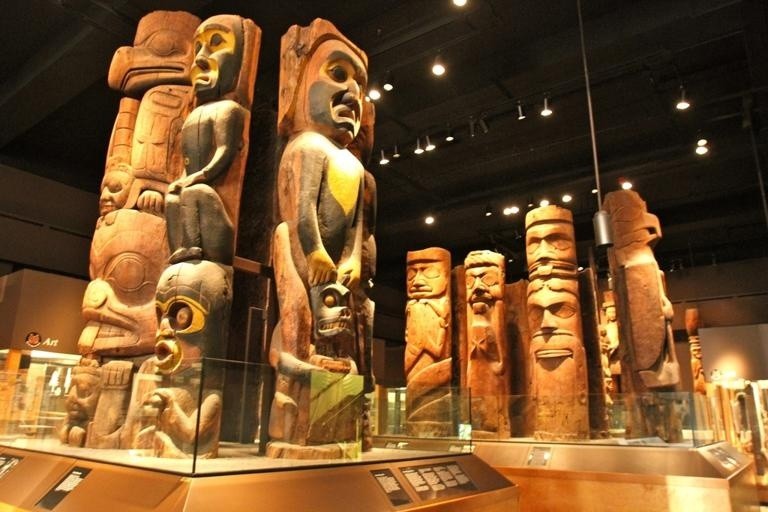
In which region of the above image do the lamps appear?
[424,134,437,152]
[482,177,632,216]
[392,143,401,158]
[431,53,448,79]
[382,69,394,91]
[515,98,526,121]
[413,136,424,155]
[378,147,391,166]
[671,79,691,112]
[479,113,491,134]
[694,126,711,157]
[445,122,455,141]
[369,82,383,101]
[539,92,554,117]
[467,115,476,138]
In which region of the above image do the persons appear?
[72,13,620,458]
